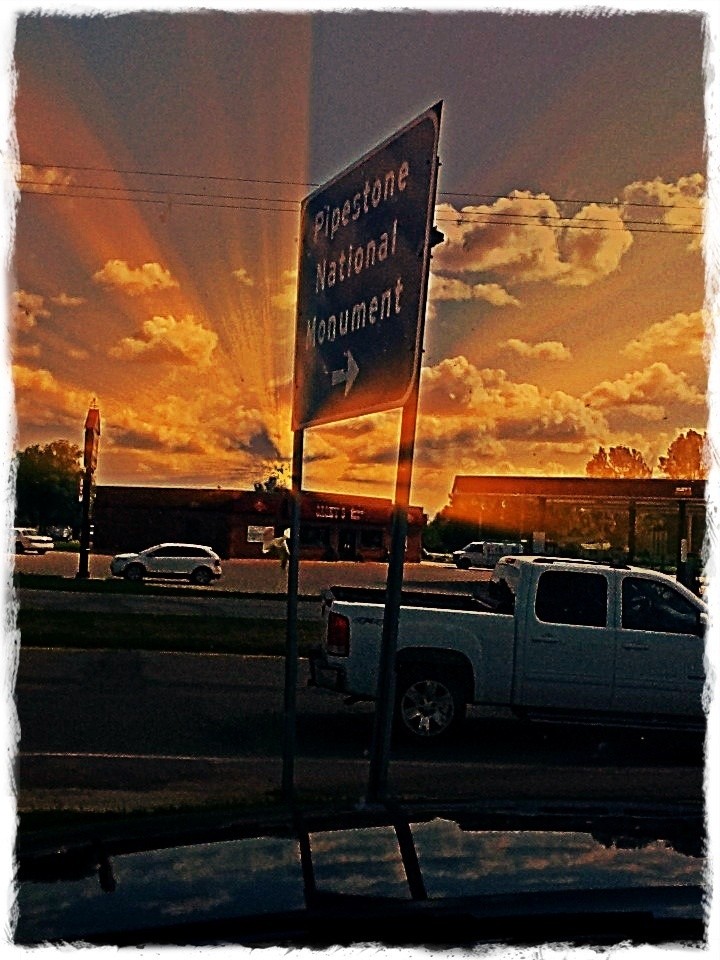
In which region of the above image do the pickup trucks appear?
[307,555,706,730]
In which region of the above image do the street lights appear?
[674,486,692,585]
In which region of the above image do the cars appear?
[14,528,55,554]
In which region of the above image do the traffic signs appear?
[287,97,443,432]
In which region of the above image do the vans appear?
[452,542,523,570]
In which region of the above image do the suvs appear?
[110,544,222,587]
